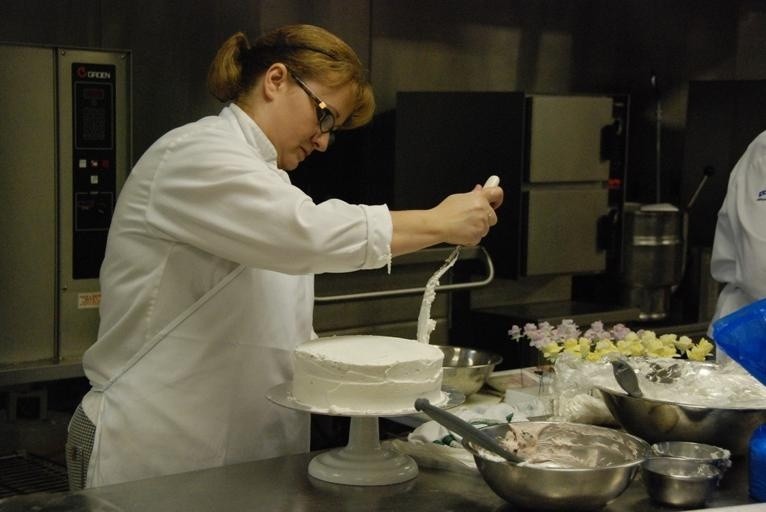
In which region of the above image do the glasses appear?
[287,66,336,135]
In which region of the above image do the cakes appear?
[291,335,446,407]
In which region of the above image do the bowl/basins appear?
[640,441,733,509]
[591,355,766,460]
[461,420,651,511]
[429,344,504,398]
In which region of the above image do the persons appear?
[61,21,506,494]
[704,129,766,358]
